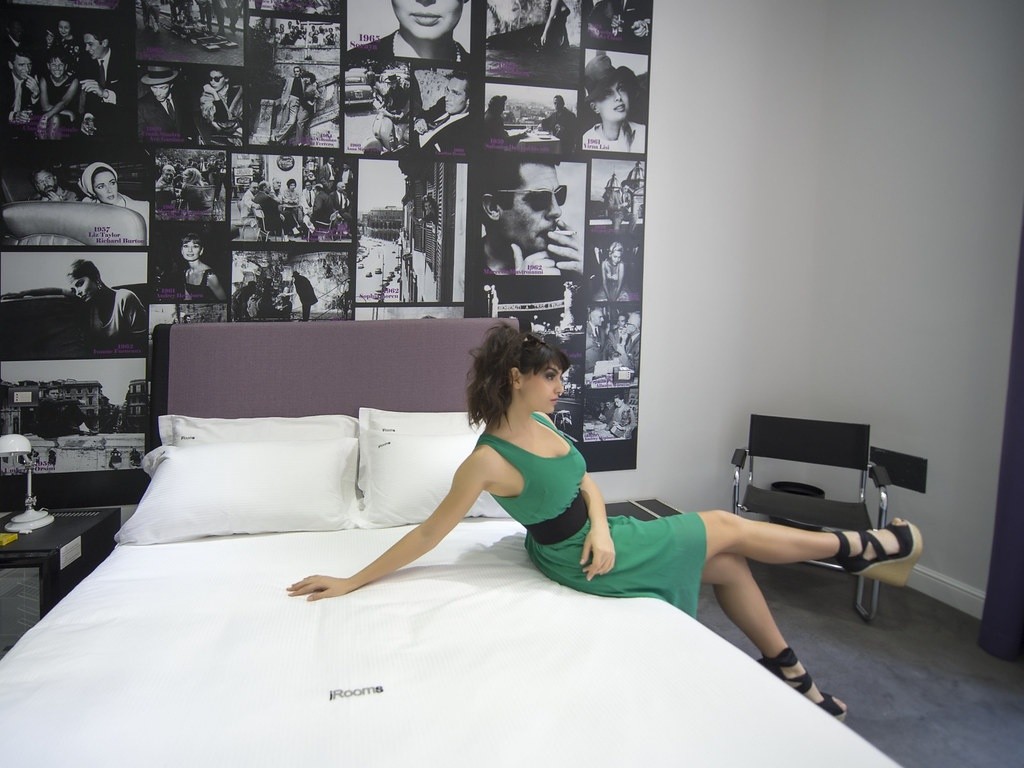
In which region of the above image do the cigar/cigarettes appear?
[554,231,577,236]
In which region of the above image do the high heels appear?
[833,520,922,587]
[762,648,847,722]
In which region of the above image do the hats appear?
[292,271,299,278]
[66,259,98,286]
[584,58,639,108]
[140,66,179,85]
[489,95,505,108]
[77,162,117,200]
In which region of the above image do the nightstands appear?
[605,498,686,521]
[0,506,121,660]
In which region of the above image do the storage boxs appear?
[613,367,635,386]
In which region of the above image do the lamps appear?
[0,434,53,531]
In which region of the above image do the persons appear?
[285,322,922,722]
[0,0,650,469]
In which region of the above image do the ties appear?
[14,82,21,111]
[99,60,105,89]
[340,192,342,209]
[310,191,311,206]
[426,114,452,129]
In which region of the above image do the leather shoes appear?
[80,429,99,436]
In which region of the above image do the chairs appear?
[231,201,283,242]
[308,209,342,243]
[731,414,892,625]
[155,186,217,222]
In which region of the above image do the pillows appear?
[356,427,512,528]
[114,437,359,545]
[359,408,486,432]
[158,415,365,511]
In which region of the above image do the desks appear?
[278,204,301,240]
[504,129,561,155]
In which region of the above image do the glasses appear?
[209,75,224,83]
[493,184,568,213]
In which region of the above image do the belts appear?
[522,488,588,545]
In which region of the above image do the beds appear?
[0,318,900,768]
